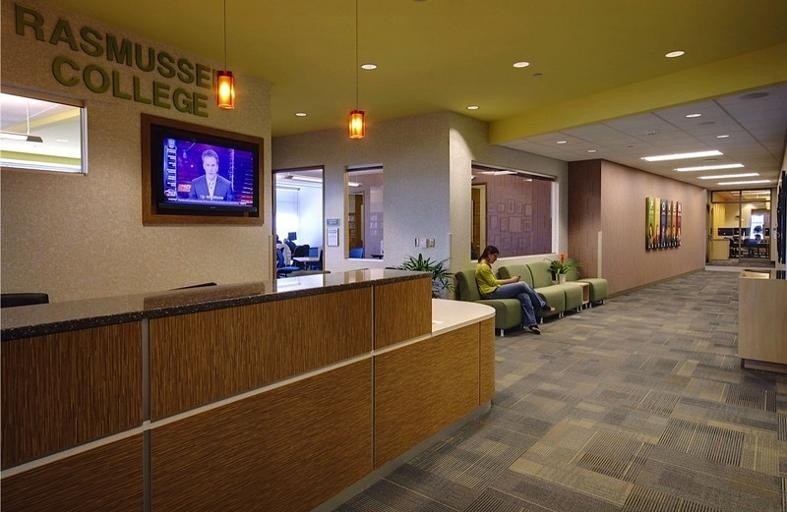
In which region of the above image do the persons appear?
[654,225,659,248]
[671,224,676,247]
[660,224,664,248]
[474,245,554,334]
[282,238,296,254]
[275,235,290,267]
[665,223,670,247]
[647,224,654,248]
[676,226,680,247]
[188,150,235,202]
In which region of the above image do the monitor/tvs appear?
[718,228,734,237]
[149,123,260,217]
[734,227,750,236]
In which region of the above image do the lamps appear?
[216,1,238,108]
[348,0,366,140]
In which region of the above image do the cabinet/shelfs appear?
[347,212,361,248]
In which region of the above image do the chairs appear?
[454,254,610,337]
[275,240,322,279]
[350,247,364,258]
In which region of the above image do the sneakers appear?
[543,304,556,312]
[527,324,541,335]
